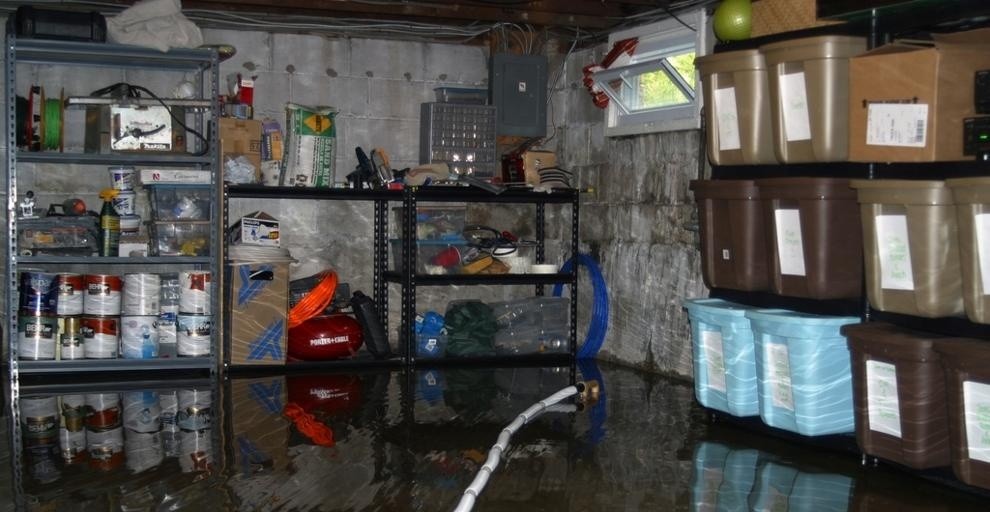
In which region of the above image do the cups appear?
[432,245,461,270]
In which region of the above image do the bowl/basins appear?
[481,241,538,275]
[415,333,449,358]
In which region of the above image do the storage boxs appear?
[838,320,948,457]
[215,117,264,183]
[745,308,862,438]
[690,174,990,325]
[19,180,214,259]
[693,35,990,166]
[391,204,571,363]
[689,299,790,419]
[930,337,989,484]
[228,210,281,248]
[690,442,938,512]
[230,375,290,475]
[225,258,291,369]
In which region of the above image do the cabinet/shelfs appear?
[11,376,226,512]
[376,182,582,367]
[375,369,577,482]
[6,34,224,379]
[690,0,990,512]
